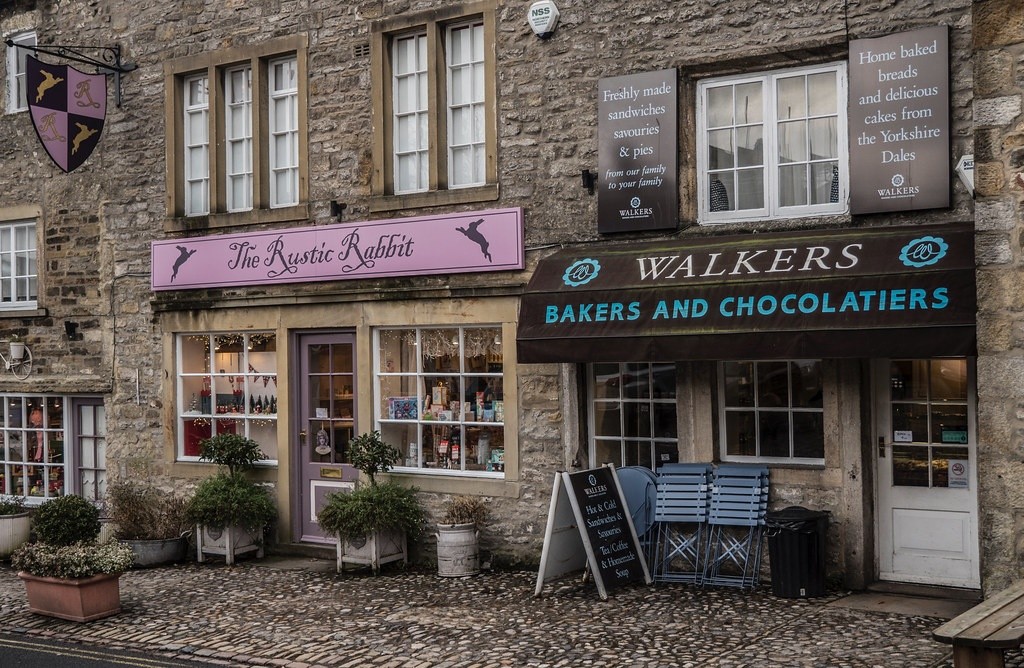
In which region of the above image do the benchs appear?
[932,579,1024,668]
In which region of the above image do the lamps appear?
[54,398,60,407]
[214,340,219,350]
[27,398,32,406]
[248,341,254,349]
[10,398,15,407]
[495,328,501,344]
[452,334,458,344]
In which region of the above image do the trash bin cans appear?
[762,505,833,599]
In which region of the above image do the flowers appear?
[11,538,140,580]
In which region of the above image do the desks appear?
[614,465,659,565]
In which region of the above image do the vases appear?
[17,570,122,623]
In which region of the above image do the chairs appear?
[654,461,770,590]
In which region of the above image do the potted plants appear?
[317,429,434,577]
[435,490,492,577]
[0,492,31,560]
[9,333,25,360]
[102,433,279,566]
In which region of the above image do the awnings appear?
[516,220,979,366]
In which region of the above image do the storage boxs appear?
[185,419,235,456]
[388,387,504,422]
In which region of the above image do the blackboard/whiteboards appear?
[562,462,648,593]
